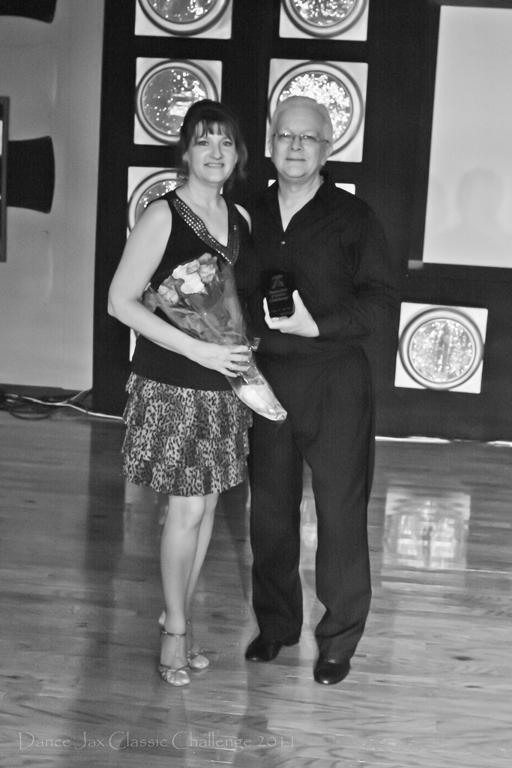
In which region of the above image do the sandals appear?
[156,613,210,686]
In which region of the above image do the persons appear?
[106,99,252,687]
[236,96,398,685]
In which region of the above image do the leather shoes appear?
[314,656,350,685]
[245,629,301,662]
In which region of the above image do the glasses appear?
[277,132,329,146]
